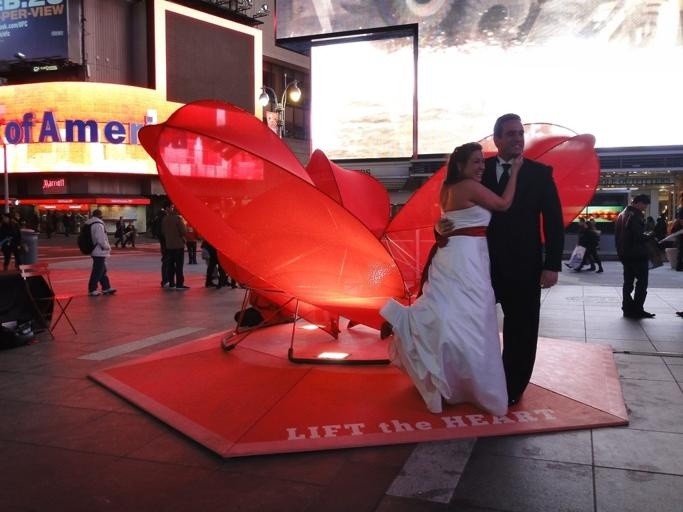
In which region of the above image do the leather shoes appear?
[508,395,518,407]
[381,321,393,339]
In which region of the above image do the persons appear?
[574,205,683,273]
[433,113,564,407]
[0,202,247,297]
[614,194,655,319]
[380,143,523,417]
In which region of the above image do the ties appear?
[496,164,512,196]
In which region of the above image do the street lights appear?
[259,79,302,139]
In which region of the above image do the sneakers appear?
[161,281,168,287]
[88,291,100,295]
[574,268,581,272]
[676,311,683,318]
[170,284,176,287]
[565,263,572,269]
[206,282,216,287]
[623,308,655,317]
[102,288,116,294]
[596,269,603,273]
[176,286,190,289]
[586,267,595,270]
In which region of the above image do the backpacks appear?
[80,221,106,255]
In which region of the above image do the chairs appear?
[19,261,80,340]
